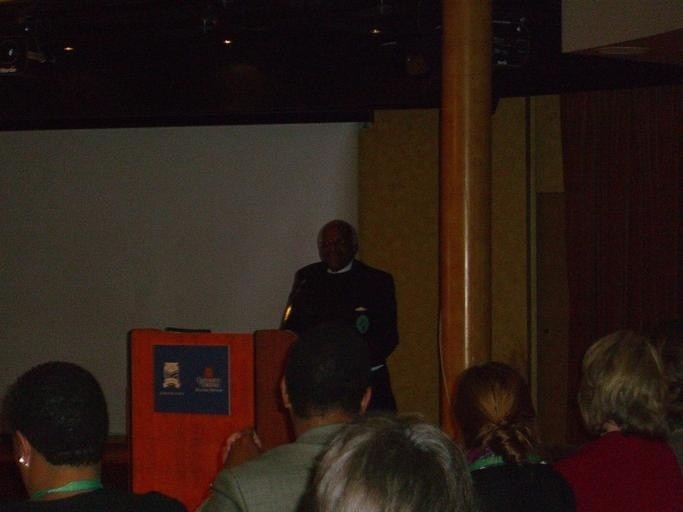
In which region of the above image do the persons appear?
[282,220,398,417]
[548,327,682,510]
[4,361,185,511]
[447,361,574,511]
[300,413,474,511]
[196,331,387,511]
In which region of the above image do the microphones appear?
[279,280,302,330]
